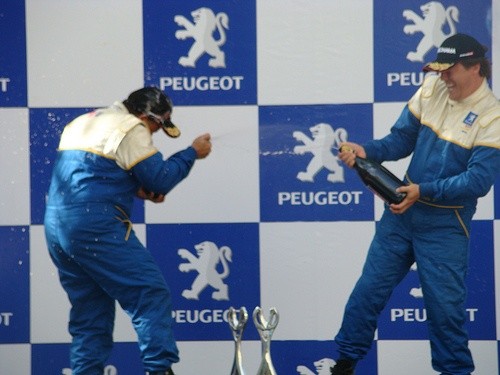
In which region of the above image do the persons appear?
[44,86,212,375]
[329,33,499,375]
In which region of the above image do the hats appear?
[422,33,485,73]
[129,87,181,138]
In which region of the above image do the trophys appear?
[253,306,280,375]
[227,306,248,375]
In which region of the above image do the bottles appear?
[338,146,410,205]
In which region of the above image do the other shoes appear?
[331,356,360,375]
